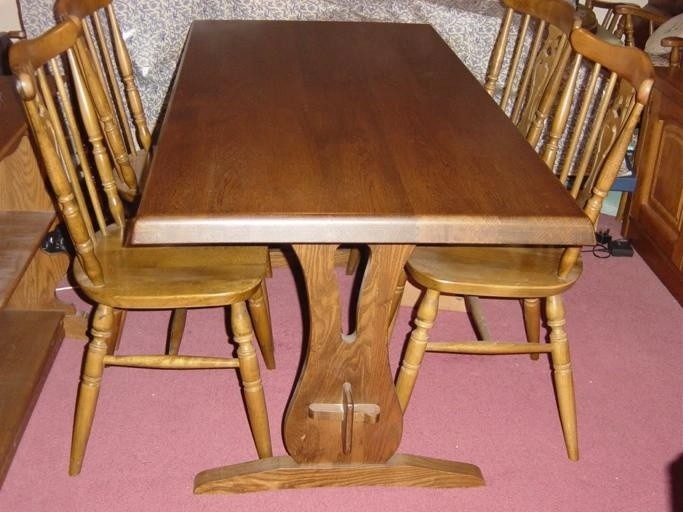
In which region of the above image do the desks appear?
[125,17,598,497]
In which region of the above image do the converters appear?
[596,232,611,244]
[609,240,635,257]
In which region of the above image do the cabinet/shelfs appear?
[0,75,91,490]
